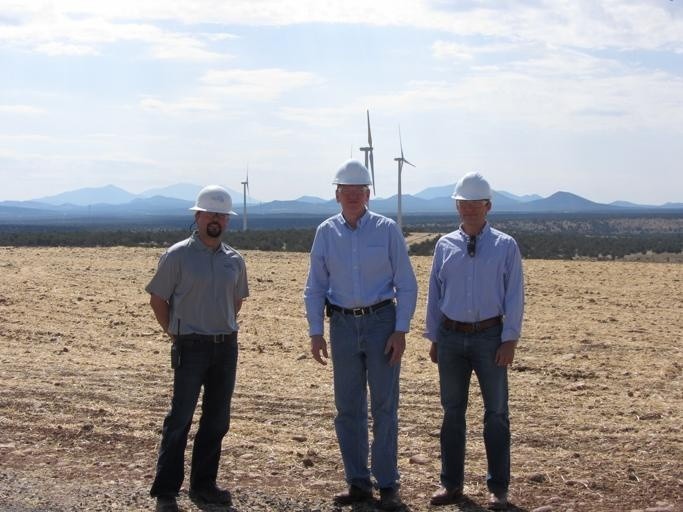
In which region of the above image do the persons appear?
[144,184,251,512]
[425,171,528,511]
[304,160,419,509]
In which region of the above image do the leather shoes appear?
[429,484,465,506]
[377,487,402,510]
[333,486,374,504]
[486,490,508,511]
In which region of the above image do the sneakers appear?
[154,493,180,512]
[188,482,232,506]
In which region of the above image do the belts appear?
[178,332,238,344]
[325,296,396,318]
[441,313,504,332]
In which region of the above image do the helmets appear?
[331,161,373,187]
[449,172,493,202]
[187,184,238,217]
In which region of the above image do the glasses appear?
[335,185,370,194]
[455,197,490,208]
[196,210,232,219]
